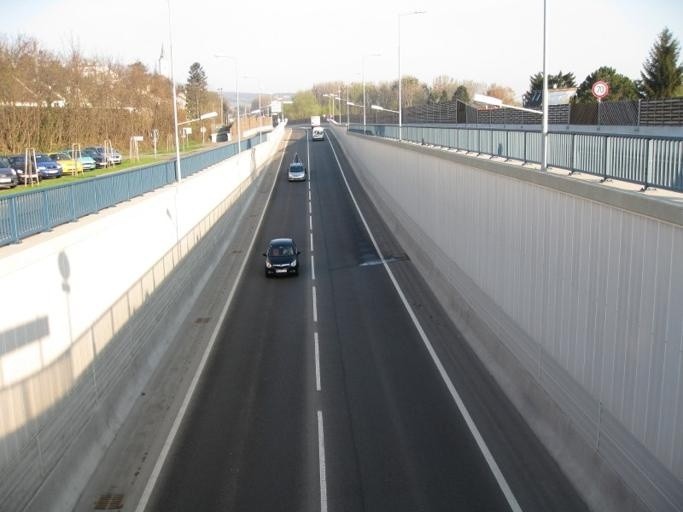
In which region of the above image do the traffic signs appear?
[592,81,608,98]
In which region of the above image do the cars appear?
[262,238,301,276]
[0,146,122,189]
[288,163,306,182]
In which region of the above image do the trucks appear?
[311,116,325,141]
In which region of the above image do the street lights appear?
[322,7,427,140]
[214,54,284,155]
